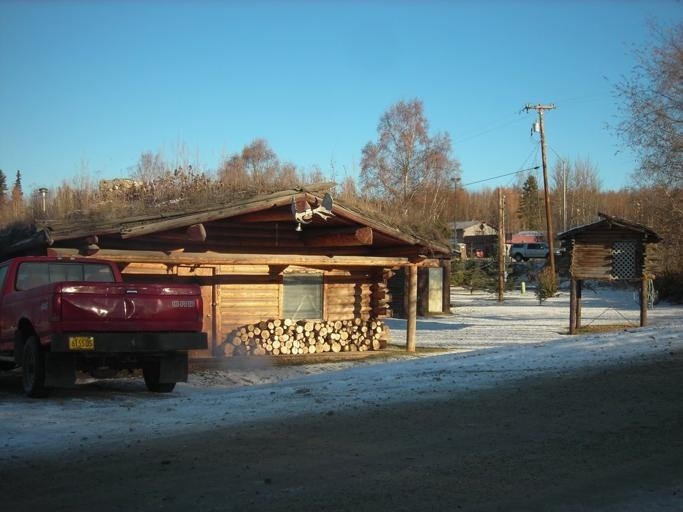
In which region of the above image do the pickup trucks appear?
[1,255,208,400]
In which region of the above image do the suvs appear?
[509,242,550,261]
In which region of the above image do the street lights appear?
[448,176,461,259]
